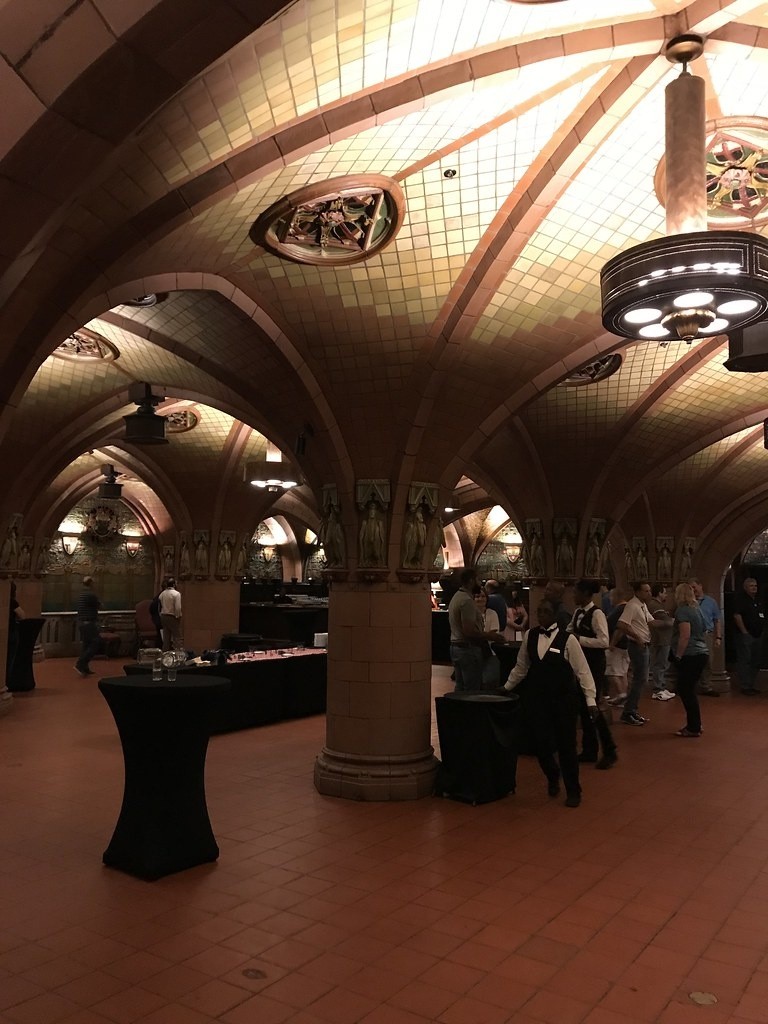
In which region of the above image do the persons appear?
[6,572,182,681]
[430,559,768,808]
[273,587,293,604]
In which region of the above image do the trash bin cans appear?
[222,633,264,654]
[434,689,522,806]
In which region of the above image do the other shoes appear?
[548,777,560,796]
[632,714,650,723]
[84,666,96,675]
[73,664,86,676]
[619,713,643,726]
[702,690,719,697]
[607,697,627,708]
[595,746,620,769]
[578,749,597,762]
[566,790,581,807]
[741,688,761,696]
[672,727,701,737]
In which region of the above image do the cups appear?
[167,669,177,681]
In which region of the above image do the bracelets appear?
[675,655,681,661]
[715,636,722,640]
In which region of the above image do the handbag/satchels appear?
[514,614,523,625]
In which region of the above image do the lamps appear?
[243,438,303,493]
[262,544,276,564]
[125,536,143,558]
[600,36,768,345]
[504,543,523,568]
[57,531,81,559]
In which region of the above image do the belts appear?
[450,642,476,648]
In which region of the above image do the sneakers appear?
[651,691,672,701]
[663,689,676,698]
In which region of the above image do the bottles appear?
[152,649,163,681]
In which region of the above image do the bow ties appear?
[577,609,586,614]
[538,629,552,637]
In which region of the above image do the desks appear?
[431,607,452,667]
[124,646,328,736]
[5,618,45,691]
[239,603,328,641]
[451,642,609,757]
[97,675,229,883]
